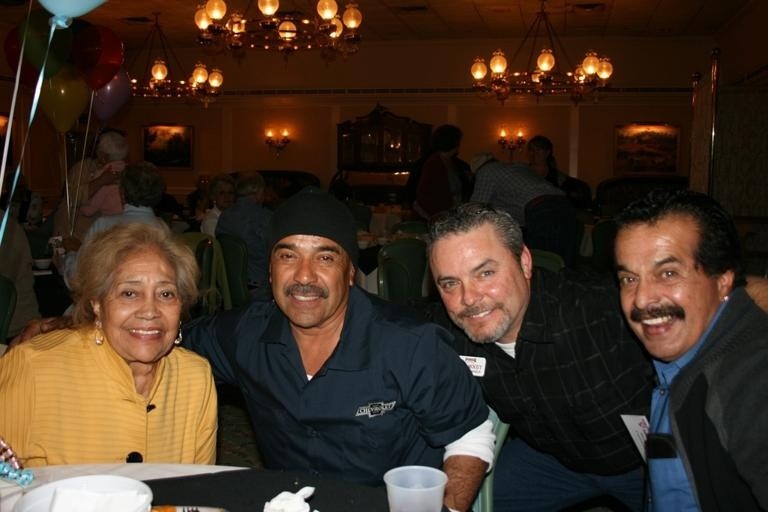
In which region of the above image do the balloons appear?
[5,0,132,136]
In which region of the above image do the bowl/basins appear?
[33,259,51,269]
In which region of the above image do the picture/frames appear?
[140,120,195,171]
[612,122,681,176]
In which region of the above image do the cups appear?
[377,237,387,245]
[382,466,447,512]
[10,474,153,512]
[357,240,368,249]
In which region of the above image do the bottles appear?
[1,436,33,487]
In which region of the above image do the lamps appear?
[498,124,524,164]
[190,1,365,66]
[263,127,291,158]
[468,1,614,108]
[124,6,224,106]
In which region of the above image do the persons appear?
[1,134,266,344]
[604,190,767,511]
[408,202,657,510]
[329,164,352,203]
[1,196,496,510]
[400,124,571,230]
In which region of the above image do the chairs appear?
[0,191,592,349]
[448,353,518,512]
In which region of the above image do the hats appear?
[470,152,494,174]
[268,192,359,272]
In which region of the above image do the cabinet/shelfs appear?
[322,99,434,232]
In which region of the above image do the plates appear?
[32,271,53,275]
